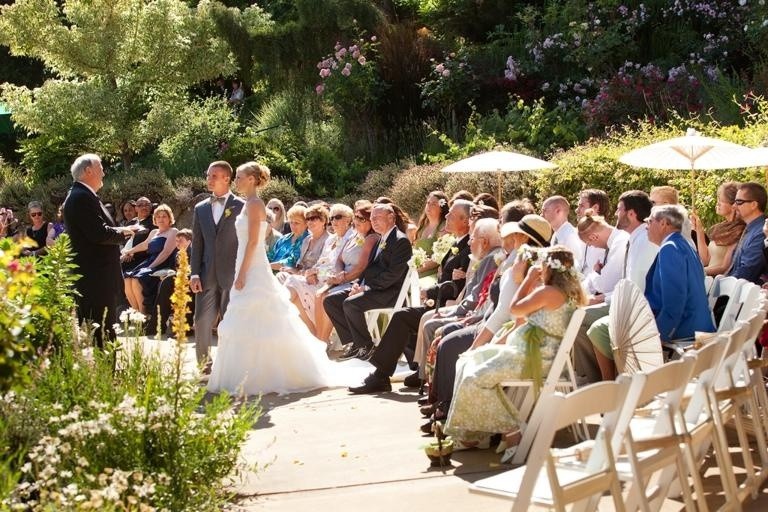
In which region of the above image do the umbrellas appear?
[607,280,662,379]
[618,128,768,207]
[433,143,560,209]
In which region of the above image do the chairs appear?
[655,320,768,512]
[720,280,761,335]
[664,275,749,356]
[544,354,697,512]
[701,310,768,441]
[704,275,713,296]
[751,288,768,320]
[629,334,740,512]
[503,310,592,465]
[365,265,421,347]
[468,373,633,512]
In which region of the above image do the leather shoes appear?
[421,422,433,433]
[349,378,392,394]
[496,441,507,453]
[500,445,518,463]
[337,345,366,360]
[356,345,376,361]
[420,403,439,415]
[404,371,426,387]
[418,395,429,404]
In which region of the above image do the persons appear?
[574,190,666,382]
[421,213,552,436]
[539,196,582,270]
[386,203,416,242]
[322,205,410,361]
[443,244,549,451]
[120,198,155,273]
[497,199,536,222]
[469,193,497,209]
[399,190,449,303]
[723,182,768,284]
[264,197,291,234]
[348,200,476,394]
[189,161,245,379]
[576,210,630,298]
[5,209,18,236]
[293,200,308,209]
[282,203,354,332]
[418,218,504,394]
[46,203,66,250]
[264,206,309,285]
[403,206,506,387]
[650,186,679,206]
[586,207,714,417]
[276,206,330,286]
[574,189,610,225]
[306,199,327,207]
[354,199,371,209]
[206,161,289,397]
[312,207,377,339]
[103,202,116,224]
[262,206,283,252]
[0,208,8,238]
[123,205,179,327]
[62,153,142,348]
[19,202,53,257]
[170,228,199,266]
[690,180,742,274]
[375,196,394,206]
[113,201,138,240]
[448,244,579,462]
[420,222,525,419]
[448,190,472,208]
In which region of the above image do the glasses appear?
[30,212,43,217]
[269,207,279,212]
[306,216,321,221]
[355,215,371,223]
[329,215,346,220]
[734,199,757,205]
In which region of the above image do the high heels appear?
[445,435,490,450]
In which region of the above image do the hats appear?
[501,213,551,247]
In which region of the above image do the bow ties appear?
[209,194,225,206]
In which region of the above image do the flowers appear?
[224,207,234,218]
[412,247,428,268]
[451,245,460,255]
[472,262,480,271]
[355,236,364,246]
